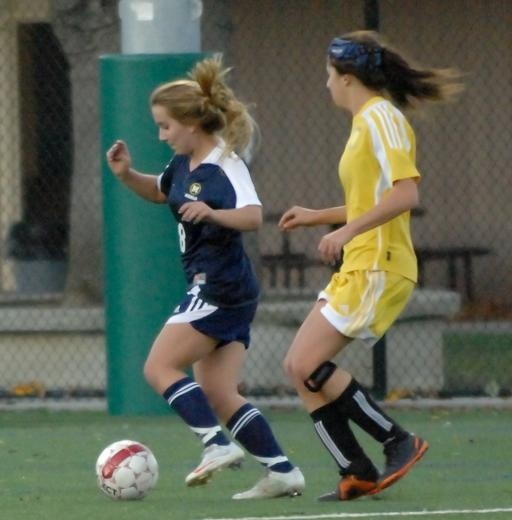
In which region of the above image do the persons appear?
[278,28,467,501]
[105,53,306,500]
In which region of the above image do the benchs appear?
[261,242,494,298]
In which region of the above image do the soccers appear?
[93,438,158,502]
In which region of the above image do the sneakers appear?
[231,466,306,500]
[380,432,429,490]
[185,441,245,488]
[317,460,381,502]
